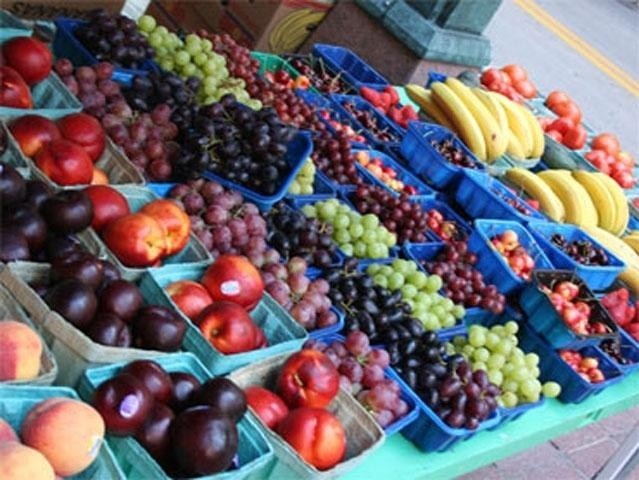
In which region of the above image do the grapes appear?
[53,9,561,452]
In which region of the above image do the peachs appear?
[0,33,350,479]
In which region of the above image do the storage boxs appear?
[144,0,333,52]
